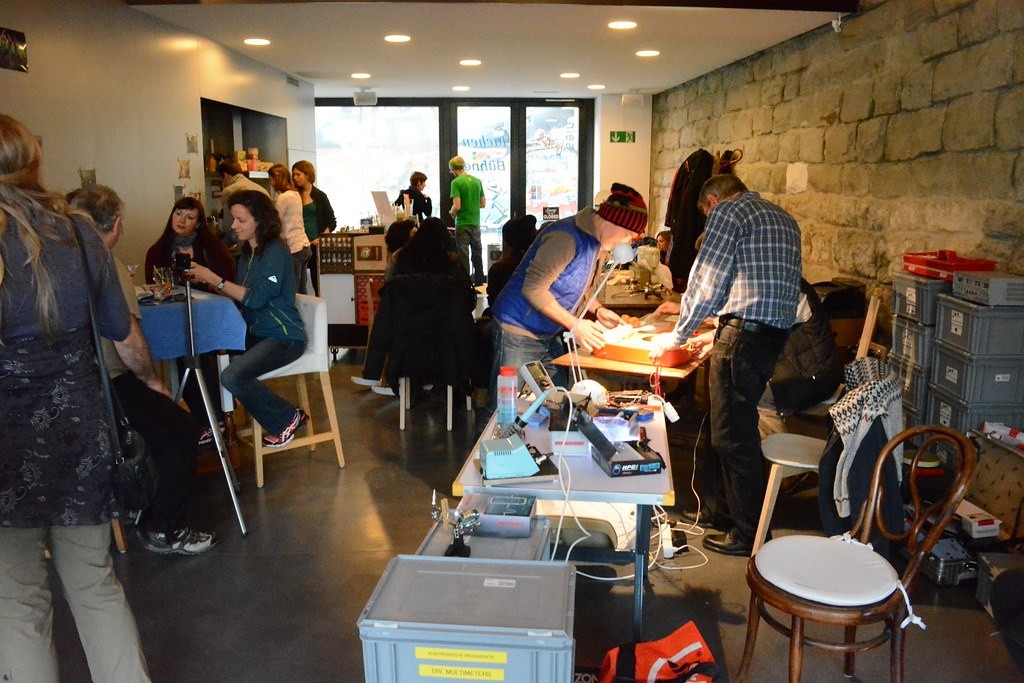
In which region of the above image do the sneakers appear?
[136,525,220,556]
[196,420,226,446]
[262,406,310,448]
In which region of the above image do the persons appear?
[65,182,222,558]
[0,109,156,683]
[392,172,432,222]
[383,217,419,255]
[215,159,272,255]
[192,189,310,450]
[376,216,479,407]
[291,159,338,295]
[648,172,805,558]
[470,214,540,410]
[448,155,486,286]
[267,162,312,295]
[757,278,843,495]
[473,182,648,442]
[655,230,671,264]
[144,197,238,295]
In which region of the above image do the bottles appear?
[496,365,518,424]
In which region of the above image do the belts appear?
[718,315,792,338]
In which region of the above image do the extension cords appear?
[659,521,676,560]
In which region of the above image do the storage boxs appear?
[975,553,1024,619]
[884,238,1024,474]
[416,511,554,564]
[356,552,579,683]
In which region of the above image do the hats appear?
[502,215,537,250]
[448,156,465,170]
[597,183,648,235]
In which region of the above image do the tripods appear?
[170,271,250,538]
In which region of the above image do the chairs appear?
[380,272,468,430]
[747,357,903,556]
[755,292,882,441]
[234,293,346,488]
[732,424,975,683]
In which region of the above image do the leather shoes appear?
[702,529,772,556]
[679,509,713,528]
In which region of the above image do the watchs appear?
[217,278,227,290]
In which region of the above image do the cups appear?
[148,265,187,291]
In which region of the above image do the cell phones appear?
[174,252,191,275]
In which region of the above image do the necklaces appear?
[301,192,310,205]
[179,246,192,254]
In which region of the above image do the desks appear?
[546,309,722,500]
[597,271,674,311]
[454,388,677,654]
[135,278,248,474]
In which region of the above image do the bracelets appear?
[594,305,604,317]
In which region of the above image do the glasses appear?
[450,171,455,174]
